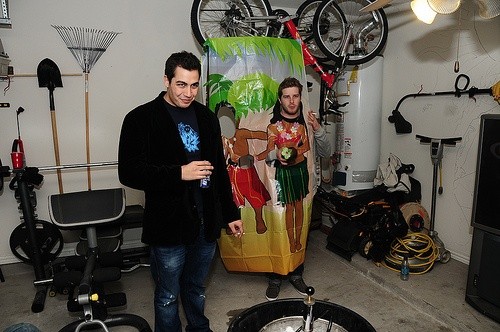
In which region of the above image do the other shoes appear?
[265,277,282,302]
[289,275,316,298]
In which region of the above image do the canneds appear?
[199,162,211,188]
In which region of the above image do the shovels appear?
[37,58,64,195]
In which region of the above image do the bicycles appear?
[190,1,388,124]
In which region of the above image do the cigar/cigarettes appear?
[232,232,246,236]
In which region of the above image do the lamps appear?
[410,0,500,24]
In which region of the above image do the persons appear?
[118,51,245,332]
[266,77,331,302]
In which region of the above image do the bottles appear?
[400,257,409,281]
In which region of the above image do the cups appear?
[10,152,23,169]
